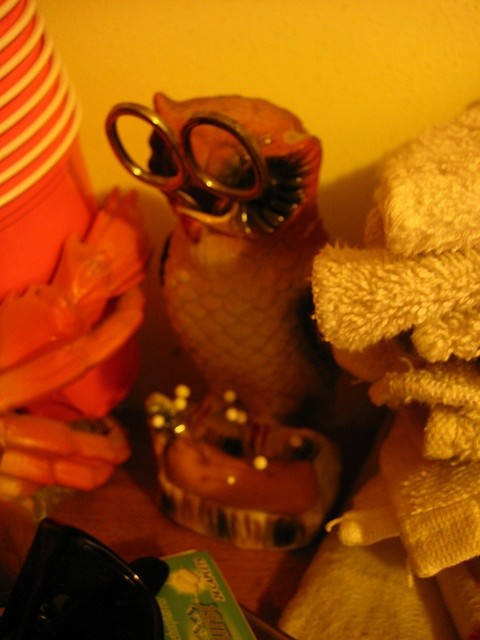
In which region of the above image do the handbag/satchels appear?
[1,518,170,640]
